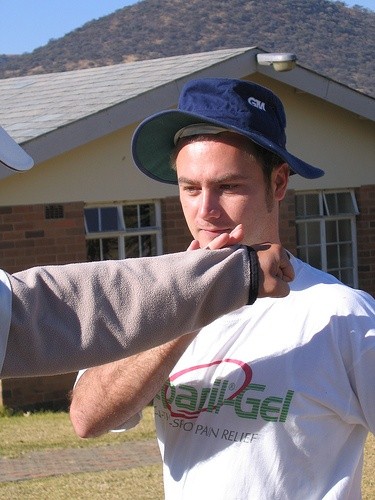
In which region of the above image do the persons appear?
[70,78,375,500]
[0,123,296,382]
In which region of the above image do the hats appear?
[0,126,34,173]
[131,77,325,184]
